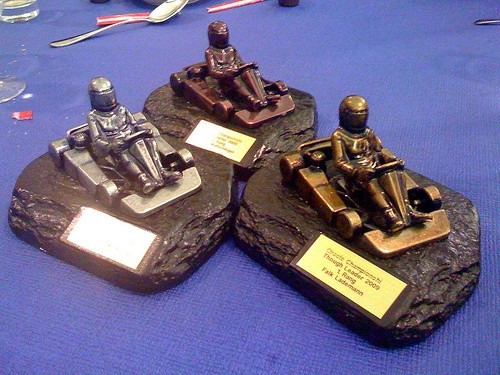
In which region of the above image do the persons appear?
[332,94,433,233]
[89,77,183,194]
[205,20,280,111]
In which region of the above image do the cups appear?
[0,0,39,24]
[278,0,299,7]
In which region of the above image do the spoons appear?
[49,0,188,48]
